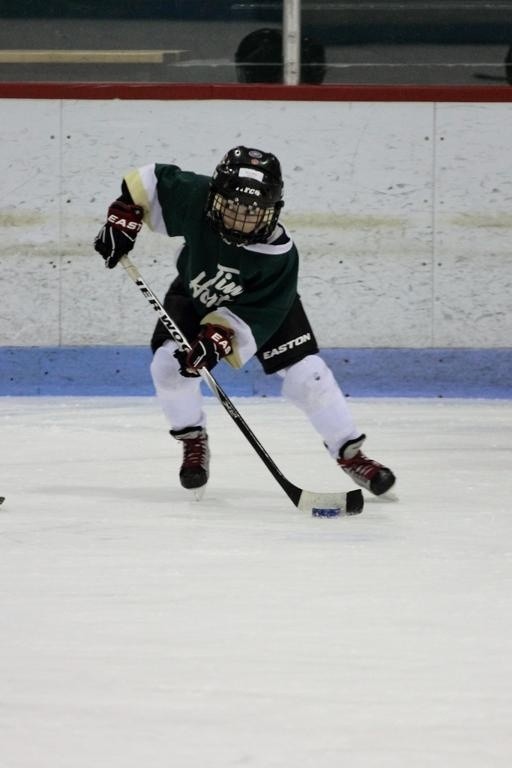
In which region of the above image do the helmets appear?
[207,145,285,208]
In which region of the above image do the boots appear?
[171,425,211,488]
[324,430,395,494]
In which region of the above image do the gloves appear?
[171,321,234,380]
[94,201,143,269]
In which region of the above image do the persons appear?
[94,146,395,496]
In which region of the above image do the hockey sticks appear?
[120,255,364,517]
